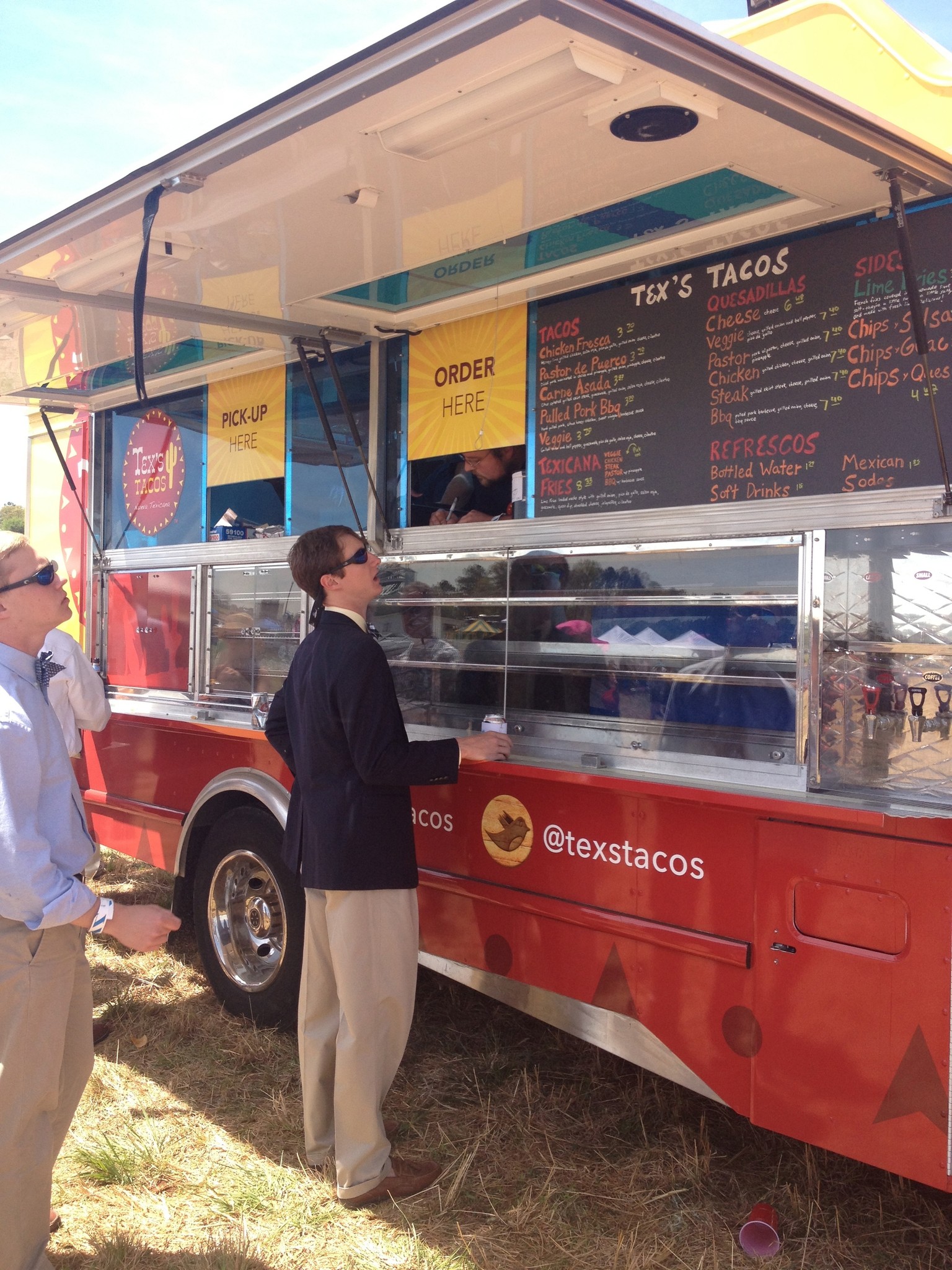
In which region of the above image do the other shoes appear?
[49,1209,61,1232]
[339,1155,442,1210]
[312,1119,398,1171]
[93,1024,110,1046]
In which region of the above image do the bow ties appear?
[36,651,67,687]
[364,621,383,640]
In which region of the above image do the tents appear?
[453,619,501,639]
[598,622,725,657]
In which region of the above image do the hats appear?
[214,613,266,647]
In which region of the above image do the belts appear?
[73,872,85,883]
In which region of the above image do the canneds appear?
[483,713,506,724]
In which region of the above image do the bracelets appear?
[491,513,506,521]
[88,896,113,933]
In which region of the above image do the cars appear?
[914,662,926,667]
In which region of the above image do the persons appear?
[389,581,464,724]
[0,524,180,1270]
[447,549,620,731]
[37,628,112,756]
[212,600,290,703]
[429,445,521,525]
[593,661,677,717]
[264,526,510,1205]
[658,591,796,763]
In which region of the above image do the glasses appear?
[324,547,370,573]
[0,560,58,593]
[457,451,492,469]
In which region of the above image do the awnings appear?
[0,0,951,565]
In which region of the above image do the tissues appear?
[210,517,248,542]
